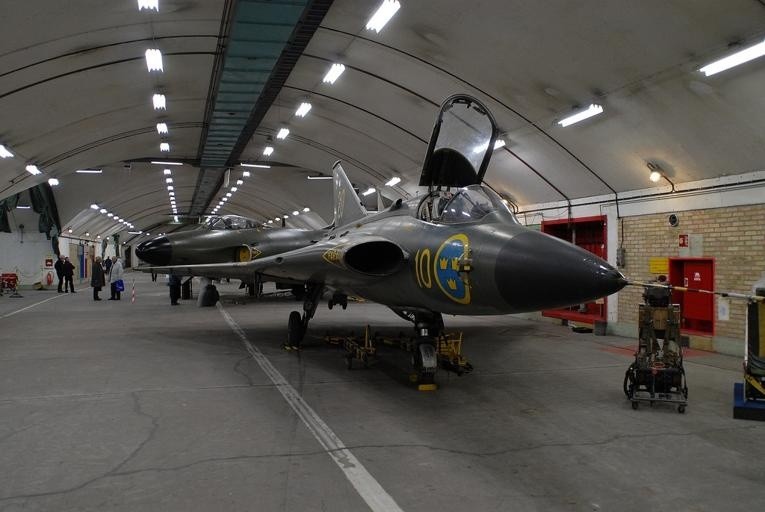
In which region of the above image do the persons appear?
[54,255,77,293]
[169,274,181,305]
[438,191,453,215]
[91,256,124,300]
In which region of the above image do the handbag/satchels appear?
[115,279,124,292]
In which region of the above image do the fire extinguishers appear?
[47,271,54,285]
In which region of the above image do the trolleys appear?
[623,279,690,413]
